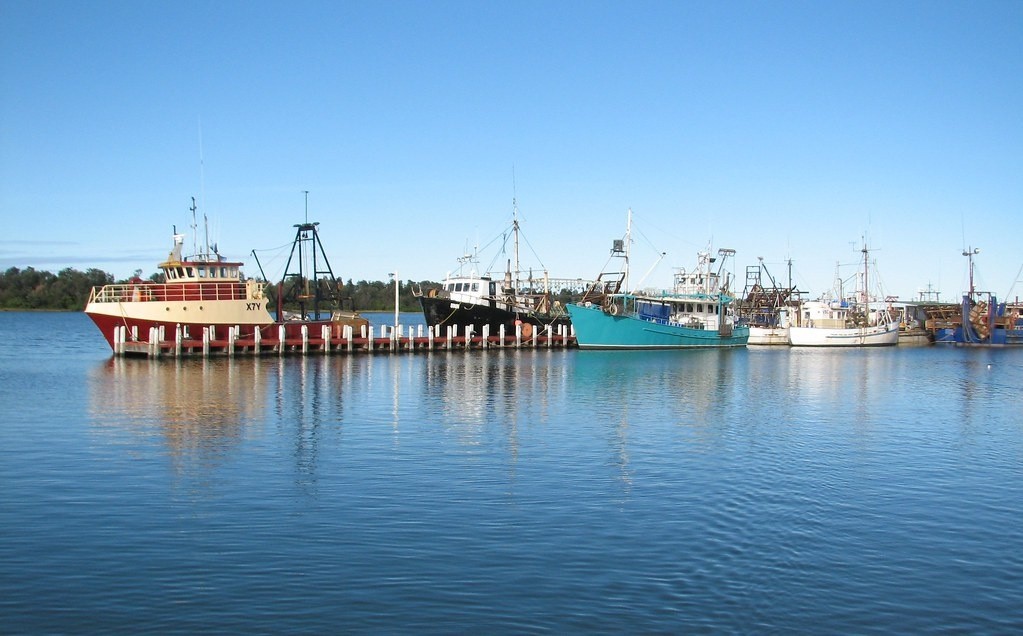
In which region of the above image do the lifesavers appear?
[129,277,144,293]
[521,323,532,336]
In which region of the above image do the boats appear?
[83,113,370,358]
[408,164,1023,351]
